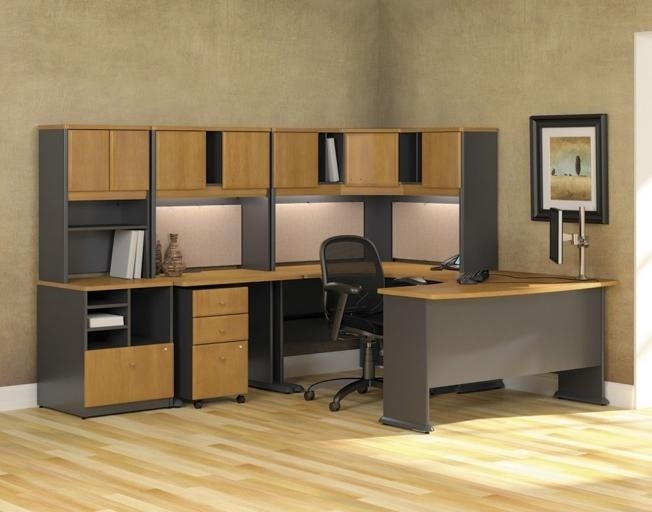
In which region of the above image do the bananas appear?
[457,269,489,282]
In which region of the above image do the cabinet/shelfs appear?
[36,280,181,420]
[40,124,153,280]
[272,124,463,197]
[174,286,249,408]
[153,127,272,198]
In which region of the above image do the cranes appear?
[326,138,340,182]
[109,230,145,280]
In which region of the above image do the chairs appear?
[304,234,427,412]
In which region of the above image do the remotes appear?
[460,278,477,284]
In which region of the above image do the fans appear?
[441,255,460,270]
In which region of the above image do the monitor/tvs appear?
[109,230,137,280]
[134,230,145,279]
[88,312,125,328]
[325,138,339,183]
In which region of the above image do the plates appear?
[36,280,250,420]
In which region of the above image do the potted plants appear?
[528,113,609,224]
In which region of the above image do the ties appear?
[549,208,563,265]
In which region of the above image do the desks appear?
[375,280,618,431]
[39,269,292,418]
[276,263,461,391]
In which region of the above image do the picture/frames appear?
[528,113,609,224]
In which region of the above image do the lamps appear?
[304,234,427,412]
